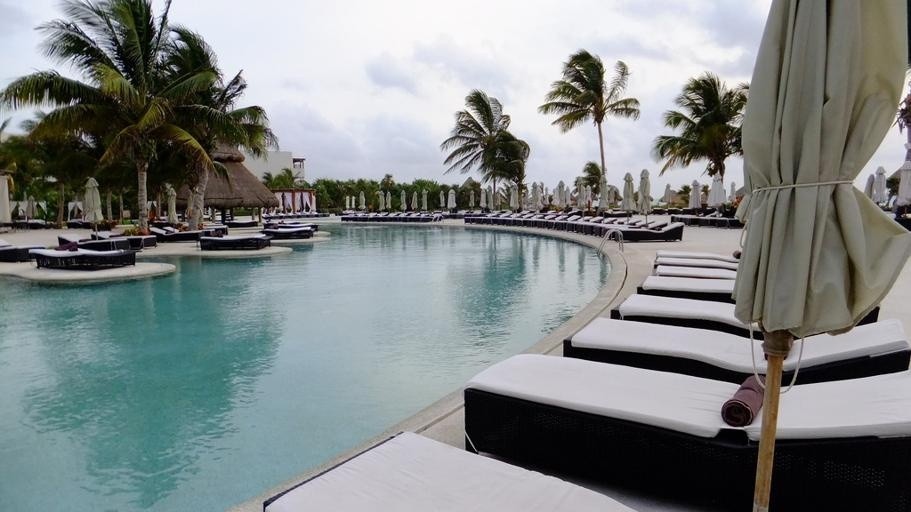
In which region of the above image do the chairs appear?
[655,256,739,271]
[58,234,130,251]
[656,264,737,280]
[564,317,911,386]
[91,232,157,249]
[263,430,639,512]
[0,239,45,263]
[464,208,745,241]
[340,212,444,222]
[29,248,143,270]
[636,276,736,304]
[463,352,911,512]
[656,250,740,263]
[148,212,330,251]
[611,293,881,340]
